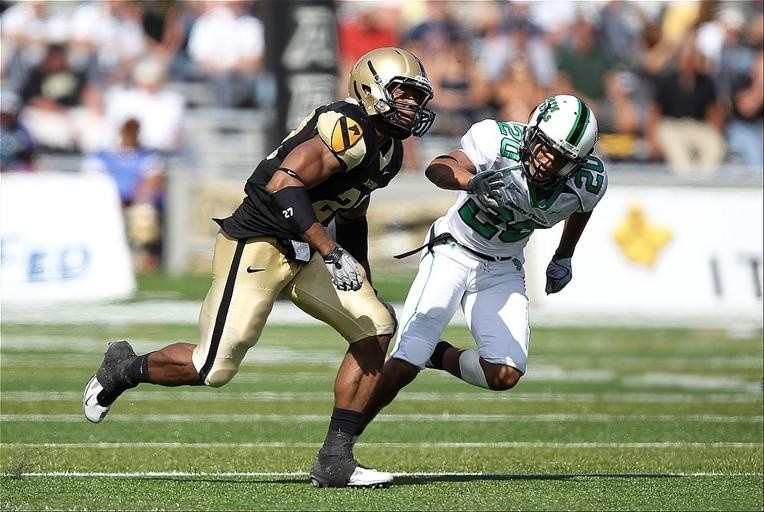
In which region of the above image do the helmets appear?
[522,95,601,166]
[347,47,435,118]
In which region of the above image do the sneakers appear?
[80,338,136,424]
[310,451,395,490]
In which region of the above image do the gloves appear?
[467,167,506,215]
[321,245,367,294]
[545,249,574,295]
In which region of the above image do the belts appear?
[392,232,513,261]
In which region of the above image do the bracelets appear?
[273,186,321,237]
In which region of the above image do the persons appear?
[351,93,607,450]
[80,47,437,486]
